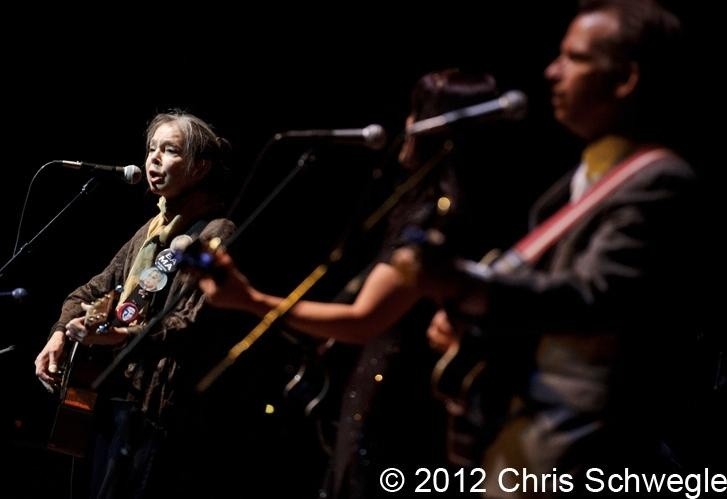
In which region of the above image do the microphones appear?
[408,89,527,138]
[53,160,141,185]
[275,123,385,149]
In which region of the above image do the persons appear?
[190,68,513,498]
[390,0,708,499]
[29,108,241,499]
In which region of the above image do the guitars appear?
[424,190,536,465]
[42,285,124,460]
[180,239,364,454]
